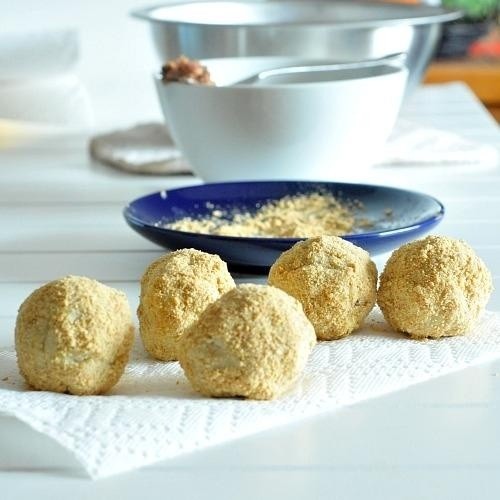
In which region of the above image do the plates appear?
[119,180,445,272]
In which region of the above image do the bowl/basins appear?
[152,54,410,181]
[129,0,467,101]
